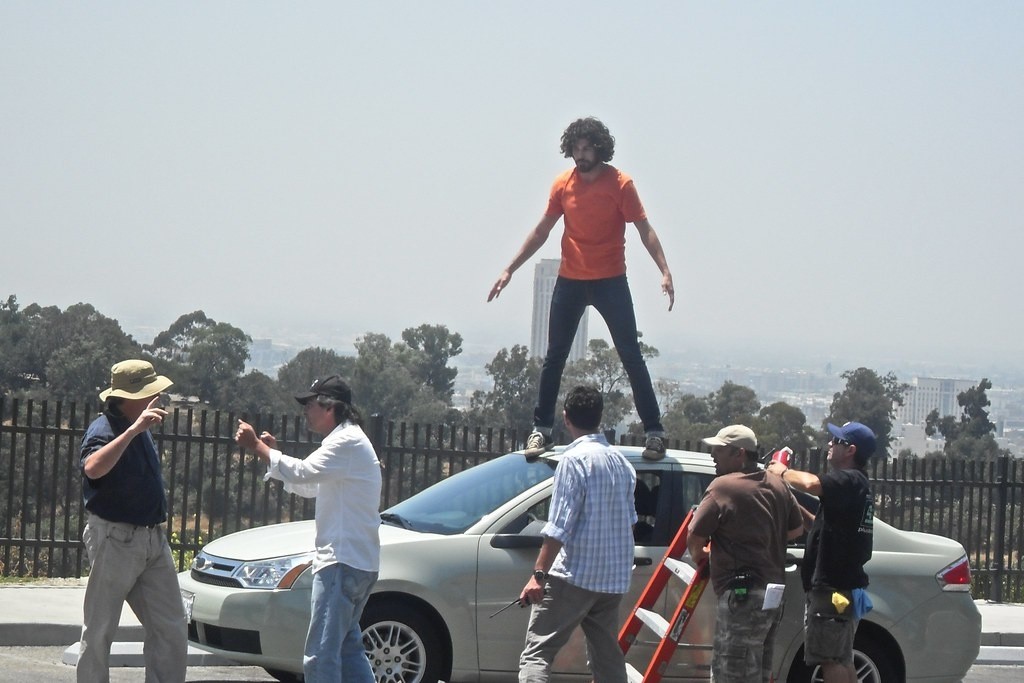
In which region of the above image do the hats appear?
[294,375,351,405]
[701,424,758,452]
[99,359,174,402]
[826,421,878,456]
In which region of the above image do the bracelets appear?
[781,468,789,478]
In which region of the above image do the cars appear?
[178,445,982,683]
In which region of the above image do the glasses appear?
[832,435,856,445]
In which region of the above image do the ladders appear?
[617,505,711,683]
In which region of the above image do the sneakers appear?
[524,432,554,458]
[642,437,665,460]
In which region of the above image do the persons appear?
[77,360,188,683]
[487,117,675,460]
[766,422,878,683]
[234,375,383,683]
[686,424,805,683]
[518,386,638,683]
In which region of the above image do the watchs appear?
[530,569,549,580]
